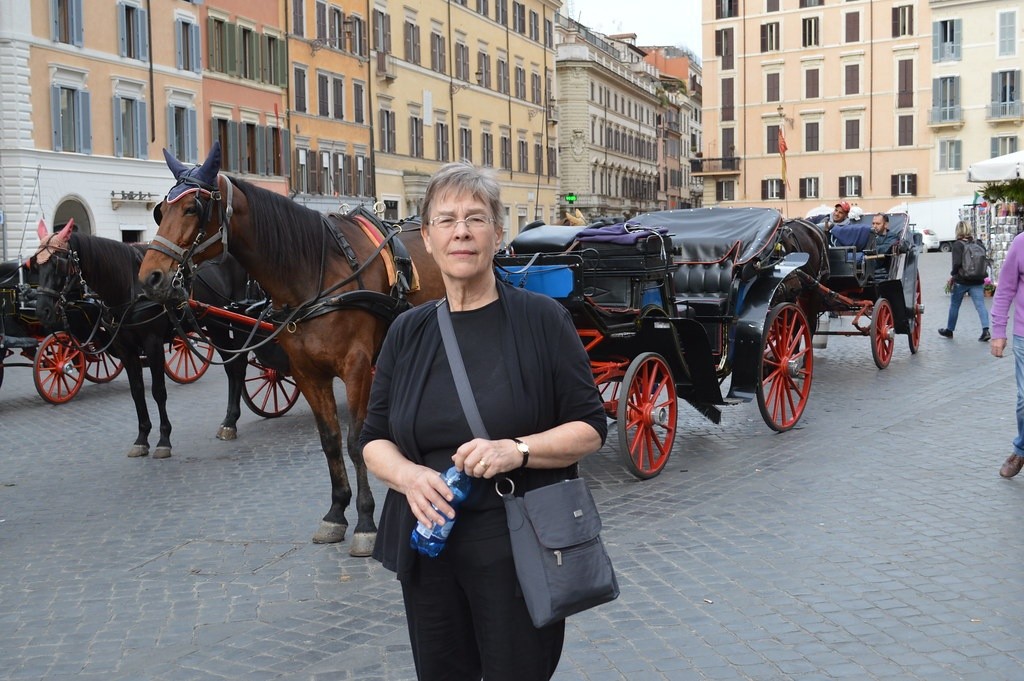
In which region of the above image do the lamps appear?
[451,69,484,96]
[777,103,795,130]
[527,95,557,121]
[310,16,353,58]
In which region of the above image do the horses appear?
[34,219,272,459]
[140,143,449,556]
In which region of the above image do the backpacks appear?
[954,240,985,281]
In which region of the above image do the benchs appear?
[669,238,738,304]
[811,212,908,275]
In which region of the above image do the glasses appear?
[428,214,496,229]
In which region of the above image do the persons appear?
[988,229,1024,478]
[939,220,991,341]
[813,201,899,275]
[359,159,607,681]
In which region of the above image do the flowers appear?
[944,276,995,297]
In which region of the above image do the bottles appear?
[410,465,474,558]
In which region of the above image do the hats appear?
[835,202,850,212]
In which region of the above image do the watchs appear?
[511,437,530,468]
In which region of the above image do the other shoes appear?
[1000,452,1024,477]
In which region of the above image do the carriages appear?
[36,217,302,458]
[774,210,925,389]
[138,141,814,557]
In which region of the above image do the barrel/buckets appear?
[811,317,831,348]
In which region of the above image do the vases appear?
[985,290,992,297]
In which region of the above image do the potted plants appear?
[690,146,704,158]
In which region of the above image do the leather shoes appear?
[938,328,953,339]
[978,329,991,341]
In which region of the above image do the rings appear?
[479,461,488,468]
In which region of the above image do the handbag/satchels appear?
[503,477,620,628]
